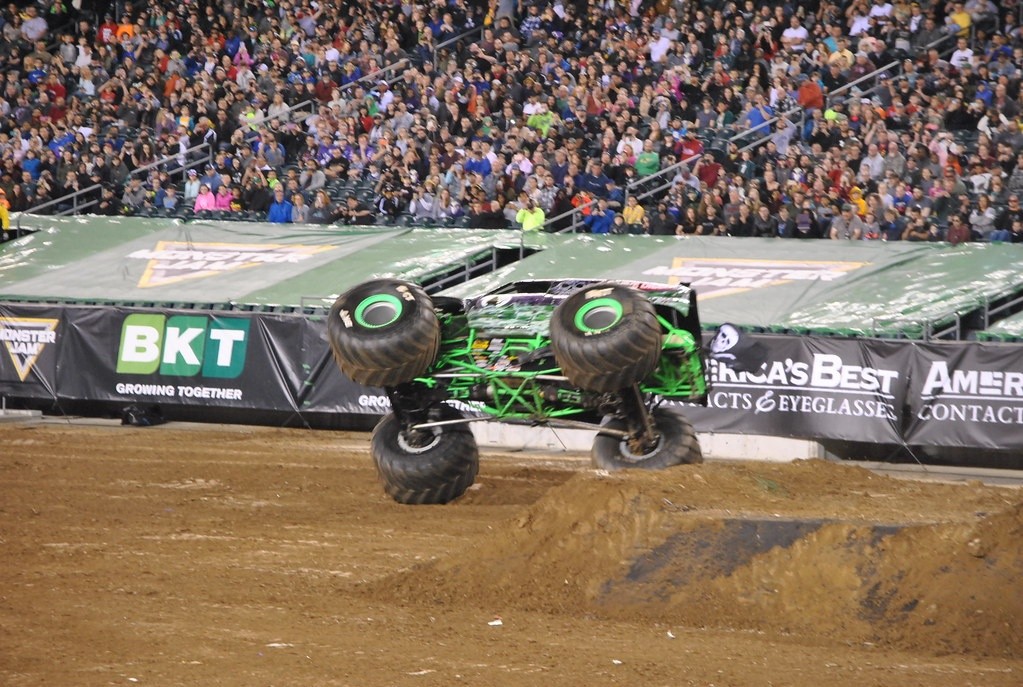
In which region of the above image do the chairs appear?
[134,165,473,228]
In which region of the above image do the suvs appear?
[332,277,715,501]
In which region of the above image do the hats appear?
[188,169,197,175]
[566,118,574,123]
[832,97,844,105]
[170,50,181,59]
[841,203,851,210]
[798,74,809,81]
[854,51,867,57]
[492,79,501,86]
[576,105,586,112]
[379,80,388,85]
[860,99,870,105]
[257,63,268,72]
[960,63,971,69]
[104,142,112,147]
[453,76,463,83]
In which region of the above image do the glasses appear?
[952,219,960,223]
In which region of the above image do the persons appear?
[0,0,1023,244]
